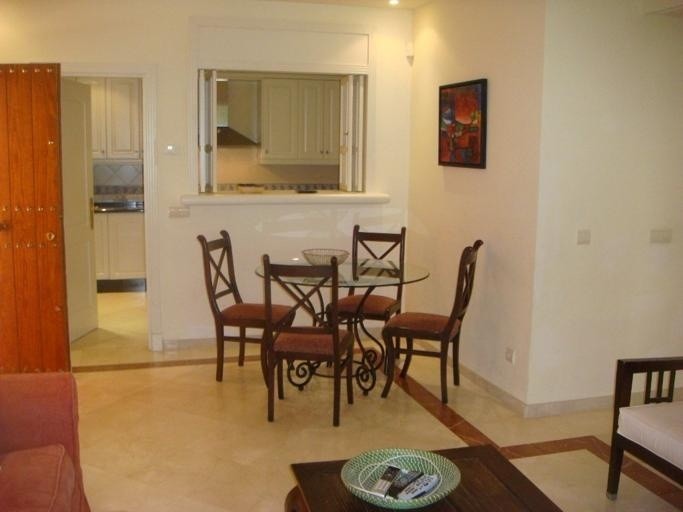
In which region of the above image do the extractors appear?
[215,79,259,148]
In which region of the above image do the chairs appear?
[261,253,354,426]
[198,229,293,380]
[325,224,404,360]
[607,354,683,509]
[381,237,483,404]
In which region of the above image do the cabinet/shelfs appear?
[228,81,301,164]
[94,211,145,280]
[300,81,340,164]
[77,78,140,157]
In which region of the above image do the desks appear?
[254,258,431,398]
[284,441,557,509]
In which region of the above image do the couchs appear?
[0,370,93,507]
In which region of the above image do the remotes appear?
[388,471,424,498]
[396,474,440,500]
[372,464,401,495]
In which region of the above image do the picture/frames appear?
[436,76,486,169]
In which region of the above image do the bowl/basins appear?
[338,447,462,510]
[301,248,350,268]
